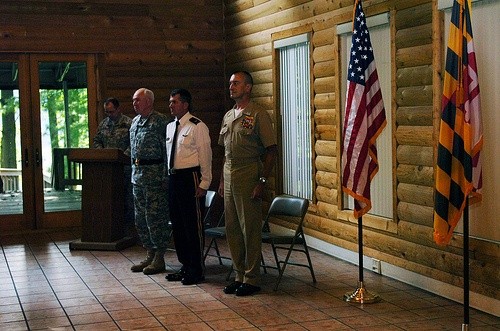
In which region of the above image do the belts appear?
[132,158,165,166]
[169,167,200,175]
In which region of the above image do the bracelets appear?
[259,177,267,184]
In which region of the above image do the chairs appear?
[168,190,233,280]
[260,196,317,294]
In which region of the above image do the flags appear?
[337,0,387,220]
[432,0,483,245]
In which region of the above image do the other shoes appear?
[142,252,165,274]
[181,271,201,285]
[165,270,185,281]
[130,250,155,272]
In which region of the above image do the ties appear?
[170,121,180,170]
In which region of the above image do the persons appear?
[129,88,169,275]
[165,91,212,284]
[93,97,137,237]
[218,69,277,296]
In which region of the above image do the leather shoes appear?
[234,283,261,295]
[223,281,242,294]
